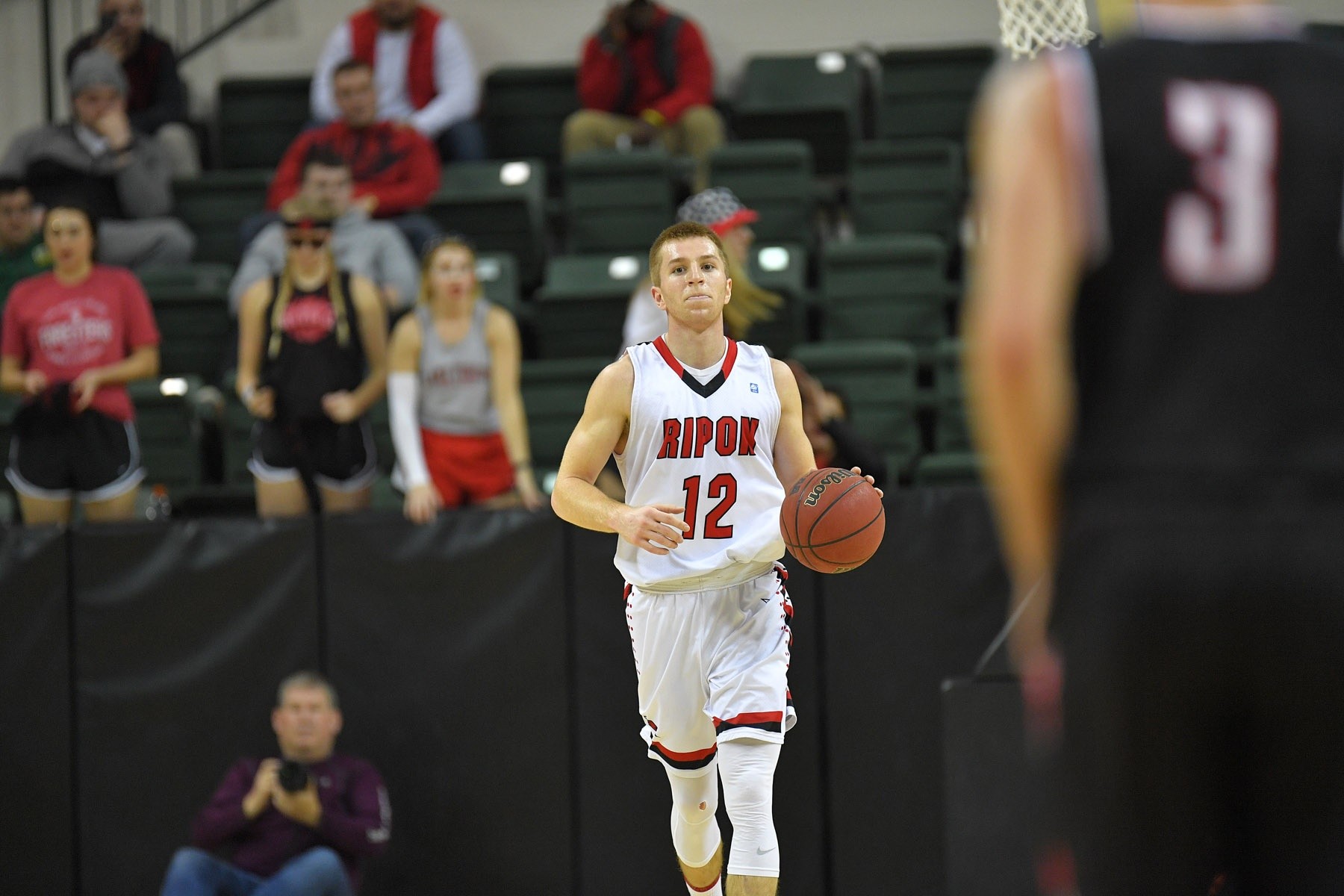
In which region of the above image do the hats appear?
[279,195,339,229]
[70,48,126,95]
[677,185,761,236]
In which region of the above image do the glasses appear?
[288,233,330,248]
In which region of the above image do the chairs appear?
[0,43,1002,525]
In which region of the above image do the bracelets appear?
[241,379,268,405]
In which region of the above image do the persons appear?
[0,0,840,524]
[966,0,1344,896]
[551,224,884,896]
[160,671,392,896]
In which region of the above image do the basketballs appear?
[779,468,885,574]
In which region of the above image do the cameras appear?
[279,760,308,792]
